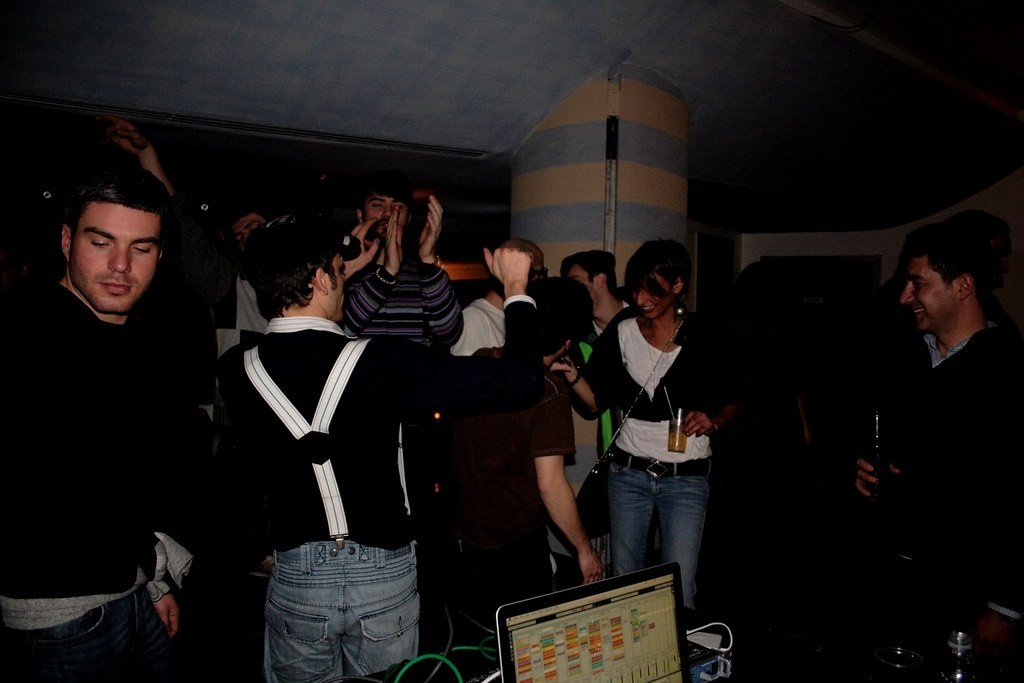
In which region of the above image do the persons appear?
[0,120,1024,683]
[550,237,744,610]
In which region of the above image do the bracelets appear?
[568,367,582,386]
[987,601,1021,620]
[376,267,396,284]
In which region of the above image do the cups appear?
[668,407,688,453]
[869,646,924,683]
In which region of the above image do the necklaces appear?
[646,317,668,387]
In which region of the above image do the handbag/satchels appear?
[576,451,617,539]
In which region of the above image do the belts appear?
[614,446,711,480]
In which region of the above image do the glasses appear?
[533,268,548,278]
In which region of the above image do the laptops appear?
[495,561,693,683]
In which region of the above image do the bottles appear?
[862,405,888,502]
[936,628,978,683]
